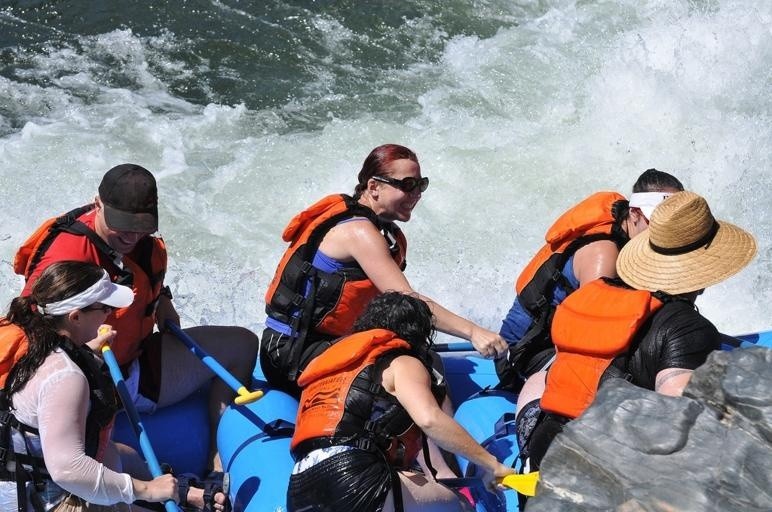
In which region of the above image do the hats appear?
[615,192,756,294]
[37,270,134,315]
[99,163,159,235]
[628,191,674,222]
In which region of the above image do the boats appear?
[90,325,770,512]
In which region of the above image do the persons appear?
[493,168,688,401]
[261,141,512,487]
[12,160,261,512]
[514,189,757,479]
[0,260,181,512]
[283,288,519,512]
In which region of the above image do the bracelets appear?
[161,284,175,301]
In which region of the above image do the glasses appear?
[373,175,428,191]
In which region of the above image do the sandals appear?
[175,477,226,511]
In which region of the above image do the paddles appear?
[436,470,542,498]
